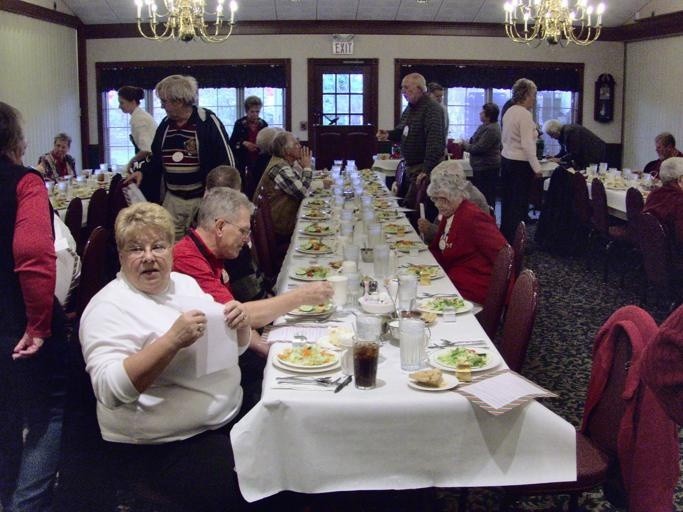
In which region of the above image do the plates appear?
[428,347,502,371]
[358,168,430,254]
[397,263,445,281]
[408,372,460,392]
[271,170,341,374]
[415,294,473,315]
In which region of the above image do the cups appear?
[585,162,651,186]
[46,162,121,198]
[331,160,432,393]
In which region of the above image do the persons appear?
[429,172,505,315]
[192,164,274,302]
[76,201,275,512]
[637,156,683,288]
[402,84,449,161]
[542,118,616,172]
[118,87,166,208]
[458,103,502,219]
[121,73,236,242]
[170,187,268,408]
[378,73,446,215]
[417,159,490,243]
[499,79,544,242]
[648,131,683,179]
[1,99,84,509]
[231,96,315,245]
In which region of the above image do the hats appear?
[426,161,466,203]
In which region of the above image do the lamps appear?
[502,0,605,47]
[136,0,237,42]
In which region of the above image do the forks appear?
[434,338,487,350]
[276,377,341,387]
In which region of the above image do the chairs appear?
[515,302,661,510]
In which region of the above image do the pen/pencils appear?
[335,376,352,393]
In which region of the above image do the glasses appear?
[223,222,250,241]
[123,245,170,258]
